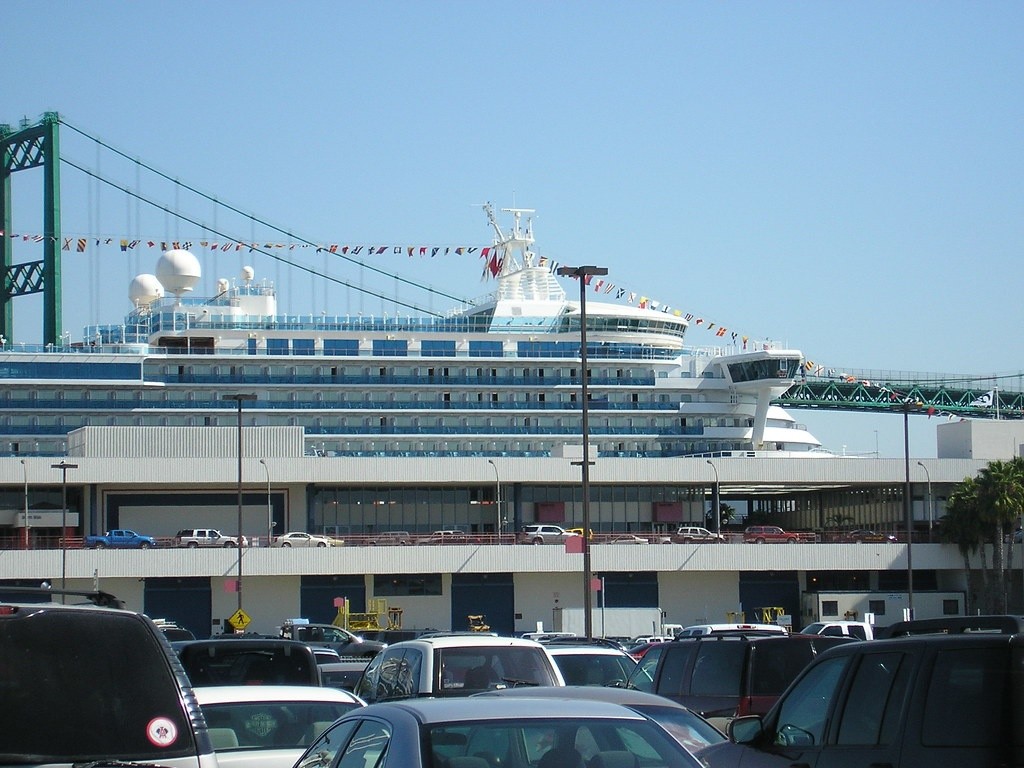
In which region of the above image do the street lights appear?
[260,459,272,547]
[488,459,502,545]
[917,461,933,543]
[221,393,258,611]
[888,400,922,622]
[21,459,29,554]
[707,459,721,546]
[556,264,608,635]
[50,463,79,604]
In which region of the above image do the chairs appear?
[304,721,333,745]
[537,747,586,768]
[468,614,489,633]
[590,750,640,768]
[464,665,499,689]
[442,756,490,768]
[207,727,239,748]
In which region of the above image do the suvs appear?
[172,528,250,548]
[677,526,725,545]
[744,525,800,545]
[518,525,578,545]
[416,530,465,546]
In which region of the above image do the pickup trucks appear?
[81,529,157,550]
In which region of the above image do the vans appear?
[361,531,412,546]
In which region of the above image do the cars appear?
[274,531,330,548]
[1004,531,1023,544]
[599,533,649,545]
[0,585,1024,768]
[849,529,898,544]
[311,535,345,547]
[563,528,595,540]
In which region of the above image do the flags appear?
[0,229,490,259]
[538,255,969,424]
[483,252,499,281]
[970,390,993,407]
